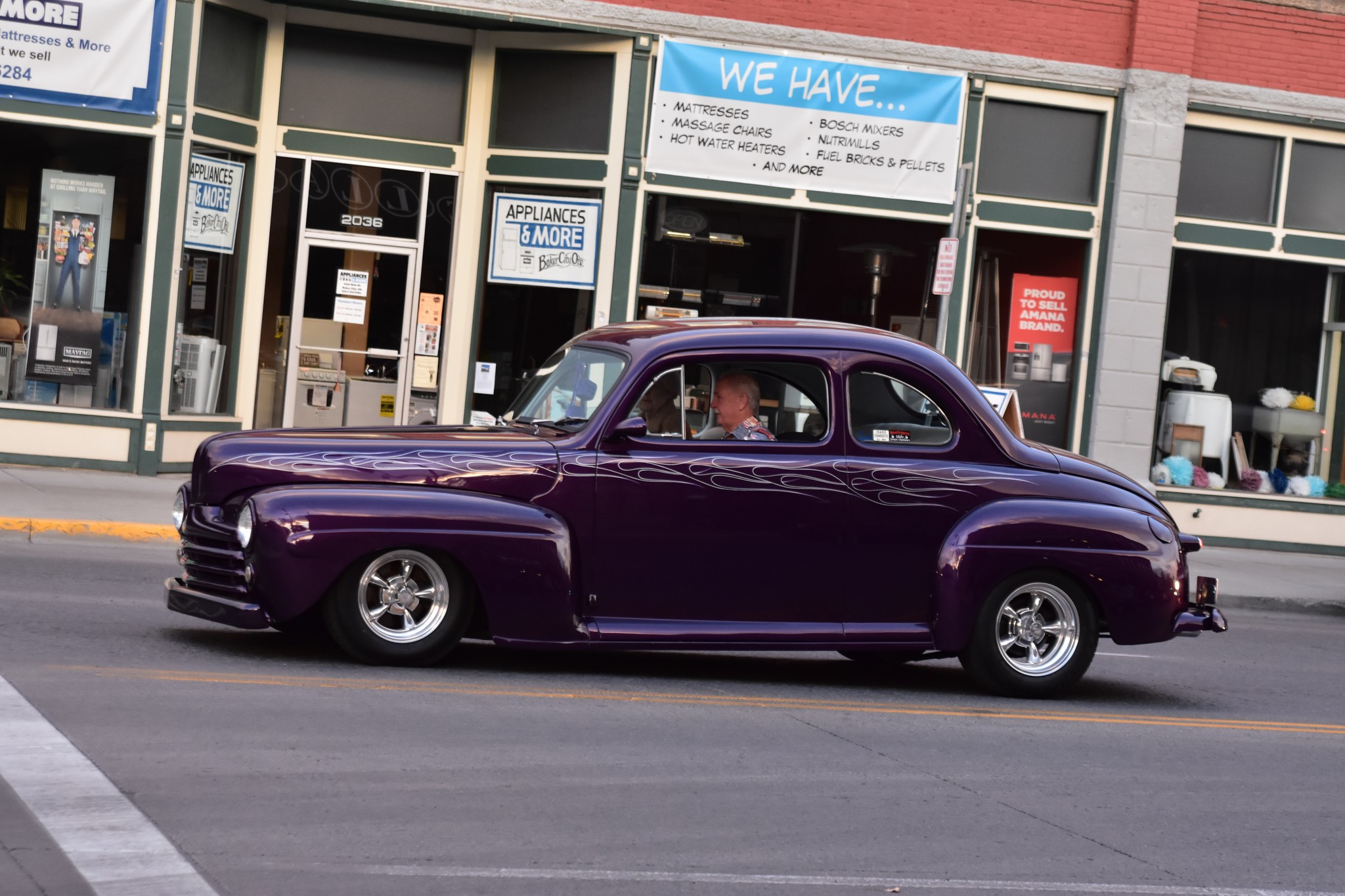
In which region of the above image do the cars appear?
[160,314,1229,702]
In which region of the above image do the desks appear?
[1250,407,1325,476]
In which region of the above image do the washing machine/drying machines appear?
[409,384,438,425]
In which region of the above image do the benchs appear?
[851,422,951,444]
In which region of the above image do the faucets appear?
[382,365,386,378]
[377,369,380,378]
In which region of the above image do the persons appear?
[710,373,778,443]
[51,214,86,312]
[639,372,694,439]
